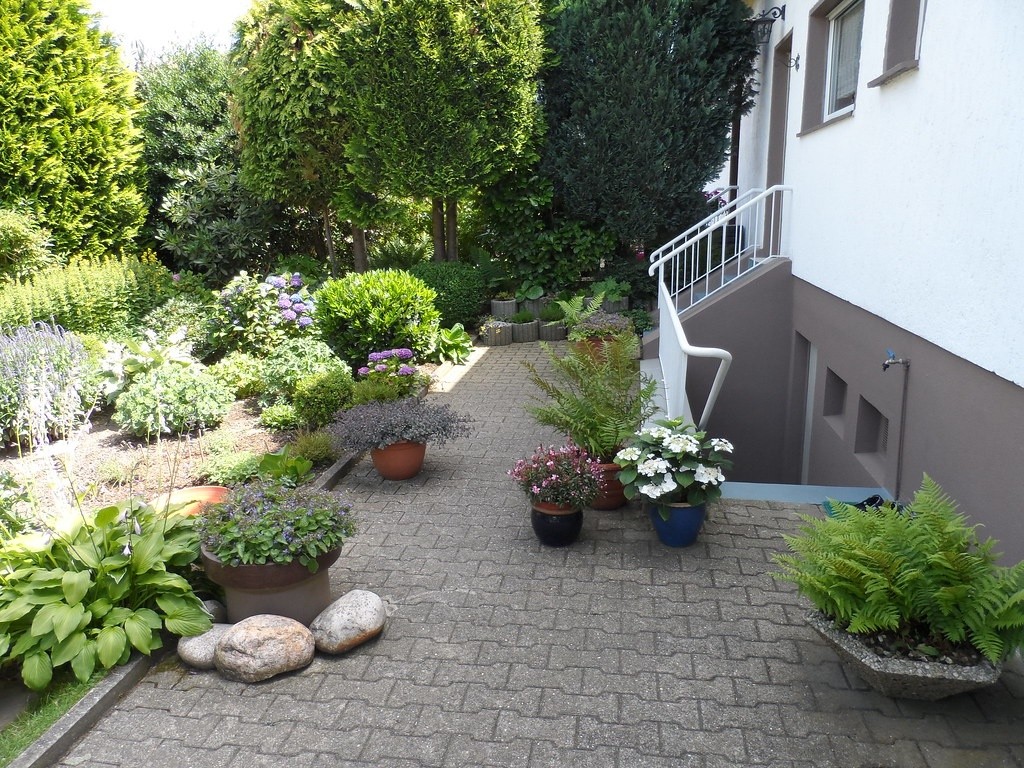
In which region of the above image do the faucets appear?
[880,348,902,373]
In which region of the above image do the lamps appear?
[752,4,786,44]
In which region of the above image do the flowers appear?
[507,439,608,509]
[325,395,475,460]
[191,479,360,573]
[612,416,735,521]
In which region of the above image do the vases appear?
[148,486,233,519]
[199,541,343,629]
[529,500,583,546]
[370,439,428,479]
[647,497,706,547]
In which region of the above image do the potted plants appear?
[768,473,1024,702]
[517,323,658,510]
[482,276,654,368]
